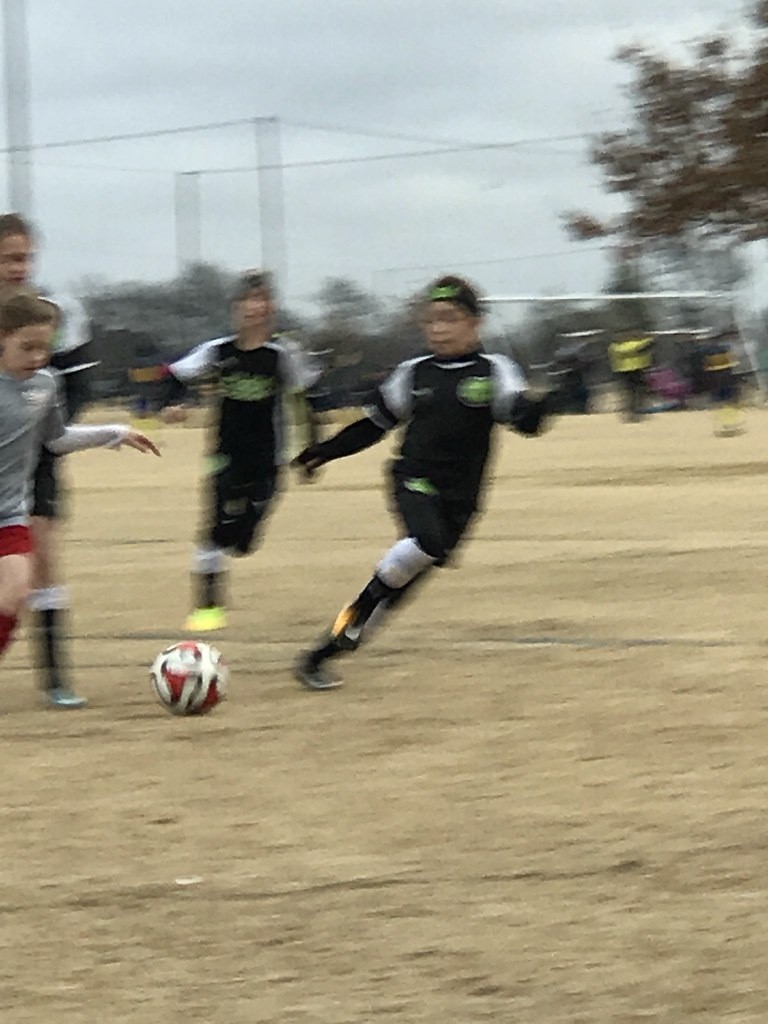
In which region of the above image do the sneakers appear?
[293,648,337,692]
[331,603,368,653]
[186,602,229,632]
[37,682,89,707]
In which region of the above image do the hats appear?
[430,275,480,317]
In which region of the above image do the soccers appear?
[149,640,228,716]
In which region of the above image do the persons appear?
[123,331,400,420]
[547,321,765,440]
[289,275,560,694]
[0,212,102,707]
[150,265,327,636]
[0,292,163,661]
